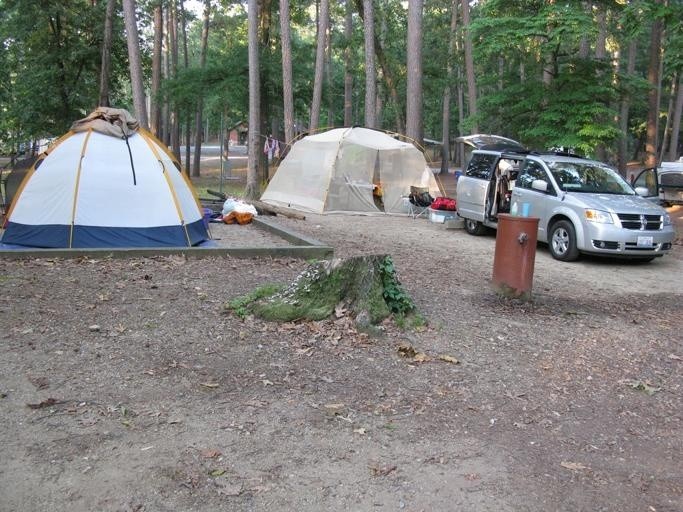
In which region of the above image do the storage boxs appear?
[428,208,457,224]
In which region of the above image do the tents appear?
[259,126,447,217]
[0,125,212,248]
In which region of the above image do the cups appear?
[522,202,529,218]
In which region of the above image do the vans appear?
[454,133,673,263]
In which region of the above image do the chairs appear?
[408,186,429,220]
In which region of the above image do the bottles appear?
[511,202,518,216]
